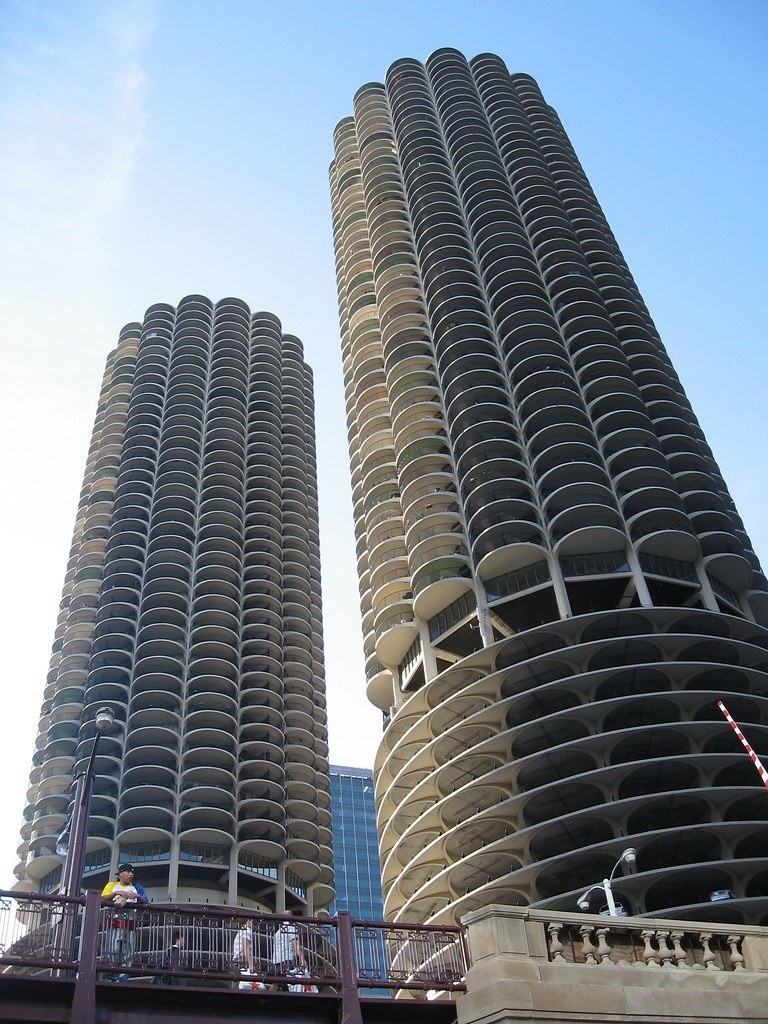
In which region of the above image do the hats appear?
[115,864,134,875]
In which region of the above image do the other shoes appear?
[104,978,113,984]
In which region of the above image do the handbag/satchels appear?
[151,961,165,985]
[286,965,319,994]
[238,968,266,991]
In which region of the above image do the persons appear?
[162,932,186,987]
[100,863,149,981]
[269,911,308,991]
[235,918,254,989]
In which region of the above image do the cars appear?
[598,900,629,918]
[710,888,734,902]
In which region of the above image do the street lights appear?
[51,706,119,979]
[577,848,636,915]
